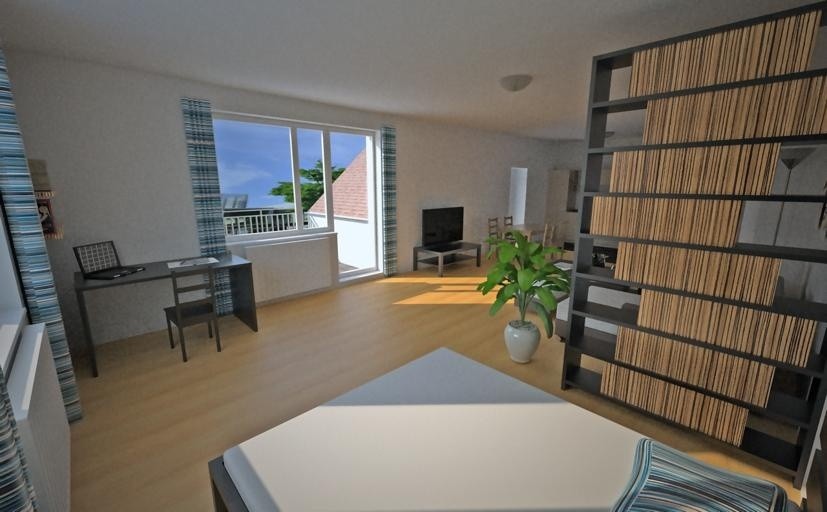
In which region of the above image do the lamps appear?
[499,74,533,92]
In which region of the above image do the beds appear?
[206,346,811,512]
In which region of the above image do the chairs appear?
[486,215,571,267]
[163,265,222,362]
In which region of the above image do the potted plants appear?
[476,227,571,367]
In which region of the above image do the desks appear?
[413,243,481,277]
[73,253,259,380]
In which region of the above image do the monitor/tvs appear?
[421,207,463,253]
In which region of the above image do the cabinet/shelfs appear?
[558,0,827,489]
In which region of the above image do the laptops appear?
[72,240,146,281]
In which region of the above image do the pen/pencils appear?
[180,259,187,265]
